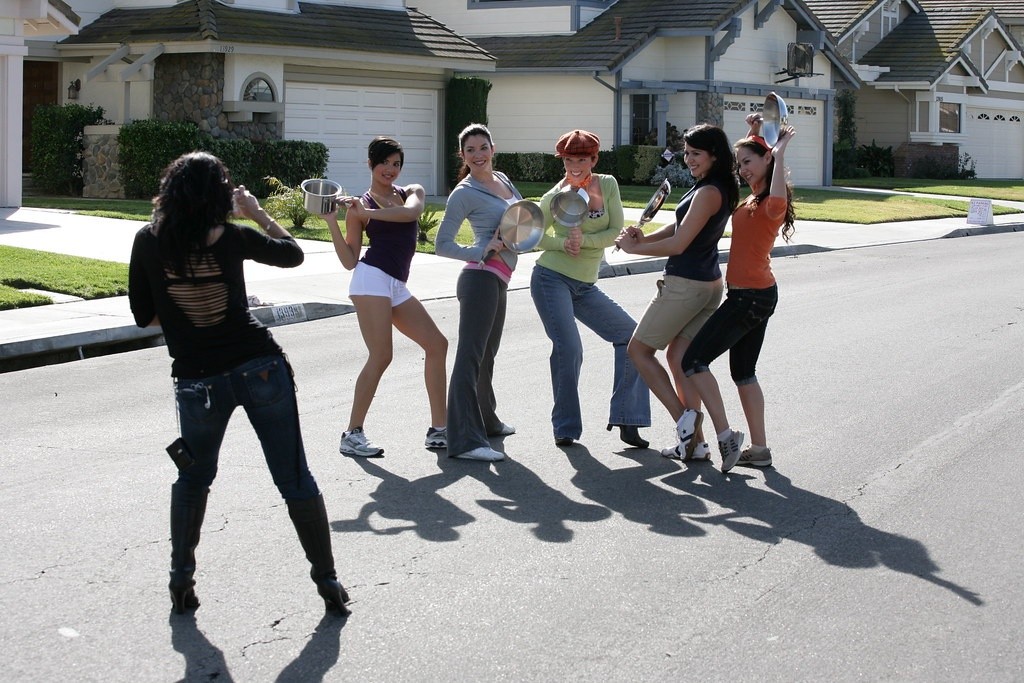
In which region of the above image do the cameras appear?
[228,195,238,213]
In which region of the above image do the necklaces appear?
[565,171,593,187]
[369,188,400,207]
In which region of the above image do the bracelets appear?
[266,219,276,231]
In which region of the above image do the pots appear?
[476,201,545,271]
[552,184,591,231]
[300,179,352,215]
[610,179,674,256]
[755,90,788,151]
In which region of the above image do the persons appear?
[531,130,651,449]
[615,124,740,461]
[680,113,795,474]
[434,123,523,461]
[129,151,351,615]
[316,136,447,457]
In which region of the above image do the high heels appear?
[317,581,349,616]
[606,424,649,448]
[555,437,573,446]
[169,576,198,615]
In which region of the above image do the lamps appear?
[68,79,80,99]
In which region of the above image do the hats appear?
[555,129,600,158]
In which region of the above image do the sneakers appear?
[339,426,384,457]
[454,447,504,461]
[736,447,772,467]
[675,409,704,461]
[661,442,711,461]
[495,423,516,435]
[425,427,447,449]
[718,430,744,471]
